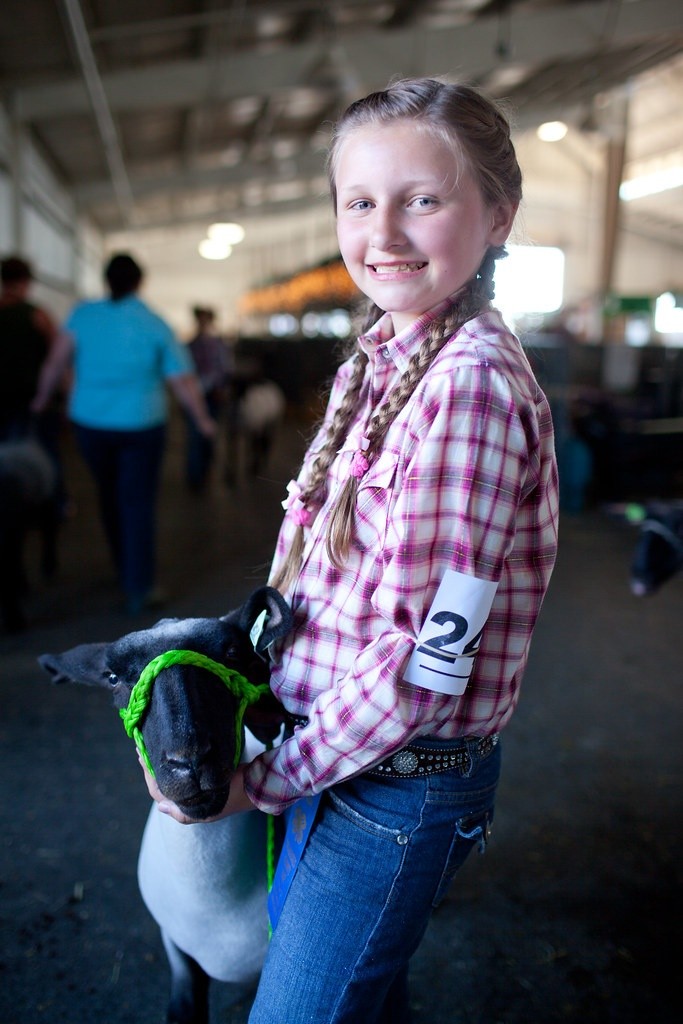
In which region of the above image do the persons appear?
[0,258,66,597]
[131,79,561,1024]
[582,343,683,596]
[31,256,232,614]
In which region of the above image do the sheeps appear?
[36,586,293,1024]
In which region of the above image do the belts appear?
[287,716,500,778]
